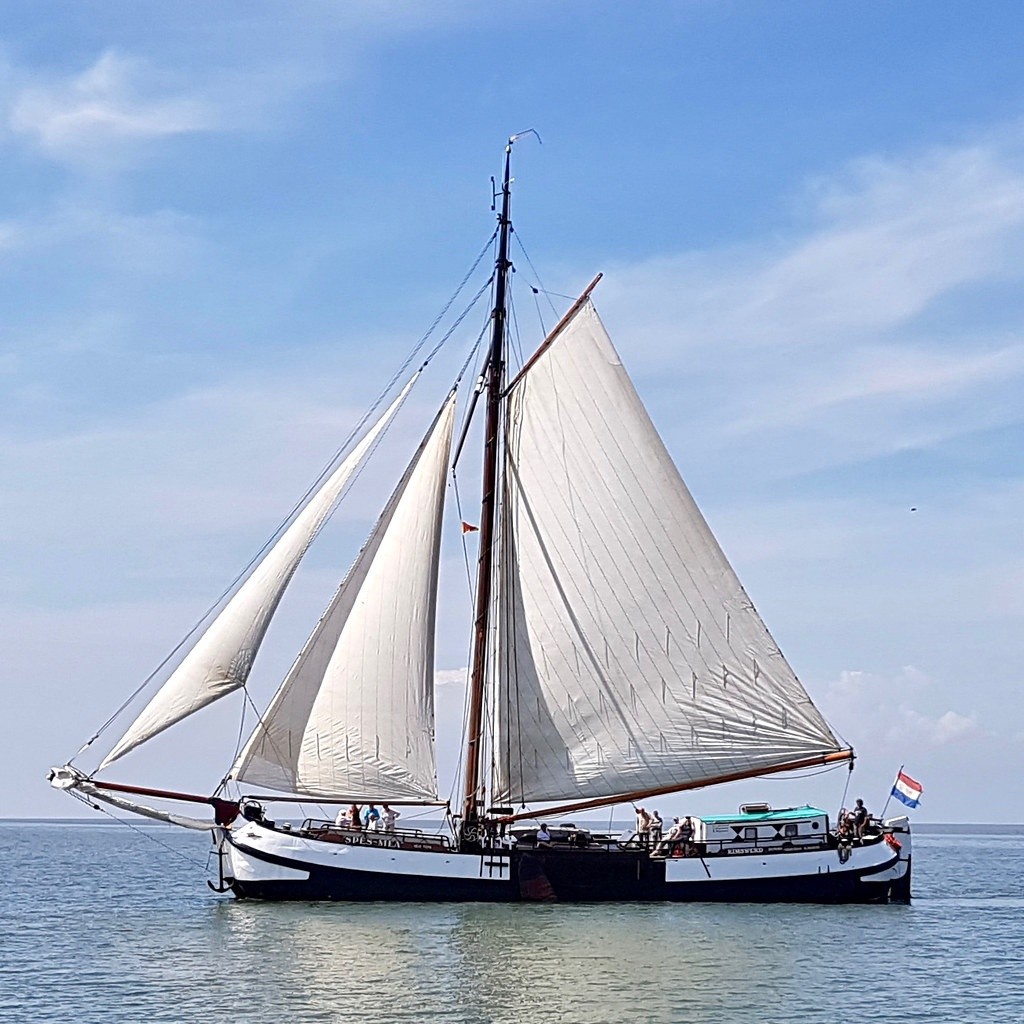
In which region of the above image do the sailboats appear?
[49,128,919,904]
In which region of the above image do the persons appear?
[650,816,695,856]
[382,805,400,834]
[839,799,884,847]
[636,808,663,854]
[536,823,557,849]
[336,804,363,832]
[364,804,380,834]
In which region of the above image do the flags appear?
[890,771,923,809]
